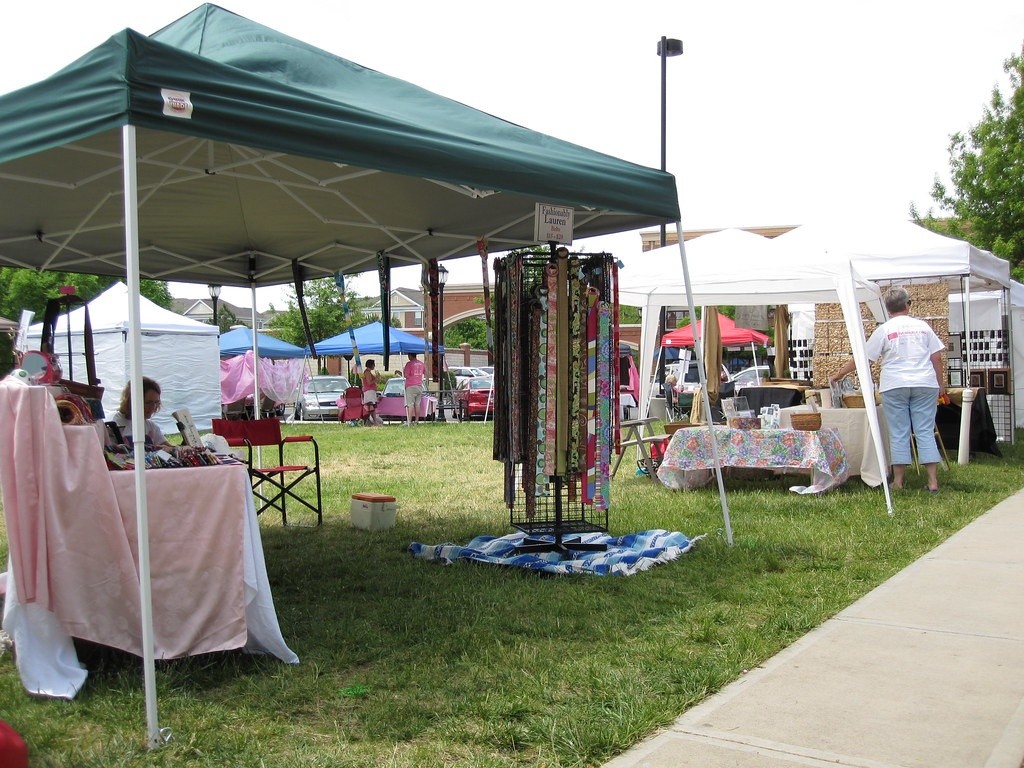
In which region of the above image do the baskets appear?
[791,412,822,431]
[841,371,876,408]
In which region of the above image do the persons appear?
[224,395,246,420]
[362,359,380,426]
[400,353,427,426]
[105,373,174,447]
[832,286,946,494]
[665,374,683,397]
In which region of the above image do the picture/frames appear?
[988,368,1012,396]
[946,334,962,360]
[964,369,988,396]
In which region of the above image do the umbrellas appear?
[653,346,679,360]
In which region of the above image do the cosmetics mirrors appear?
[19,349,53,384]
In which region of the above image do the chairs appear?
[620,394,637,421]
[337,387,364,423]
[211,418,322,528]
[224,394,250,421]
[250,396,277,419]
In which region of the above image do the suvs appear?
[650,360,736,417]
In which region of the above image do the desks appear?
[105,451,301,665]
[737,388,802,417]
[777,405,893,488]
[375,396,438,422]
[935,387,1005,457]
[656,425,851,495]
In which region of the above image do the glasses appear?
[906,300,912,306]
[144,400,161,407]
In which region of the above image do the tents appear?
[18,280,222,436]
[768,204,1016,447]
[218,326,305,423]
[305,321,461,427]
[647,311,771,415]
[610,225,928,518]
[0,2,734,753]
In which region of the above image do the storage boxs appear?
[350,494,399,532]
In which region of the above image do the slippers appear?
[924,483,939,493]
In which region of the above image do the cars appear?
[733,365,794,398]
[294,375,352,421]
[374,377,435,421]
[443,366,494,422]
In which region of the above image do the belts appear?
[492,246,622,519]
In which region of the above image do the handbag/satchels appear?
[39,295,104,420]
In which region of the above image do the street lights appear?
[655,34,685,250]
